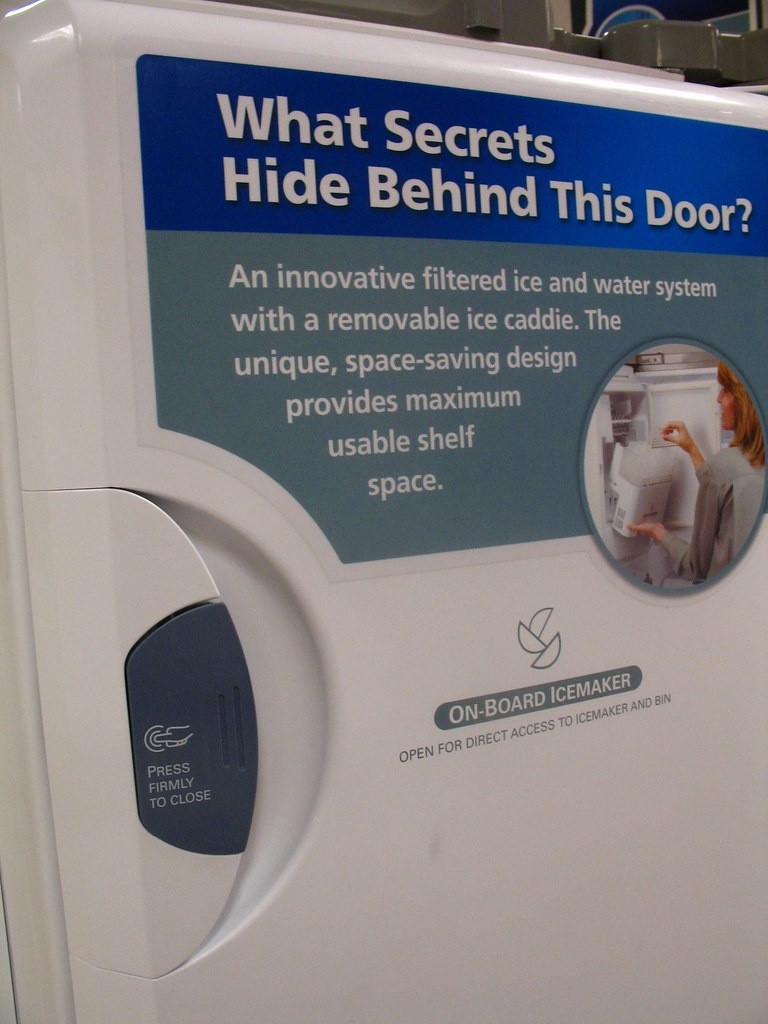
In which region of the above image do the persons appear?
[627,358,766,584]
